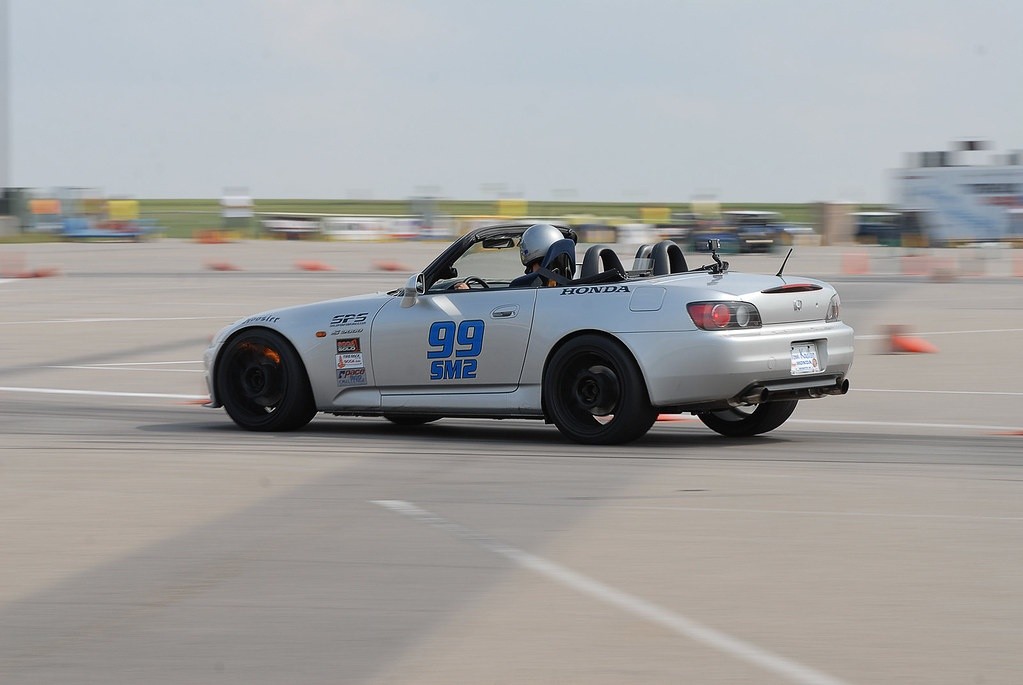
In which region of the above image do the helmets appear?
[519,224,565,265]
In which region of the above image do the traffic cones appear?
[883,321,937,356]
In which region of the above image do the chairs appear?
[631,244,654,275]
[529,239,575,286]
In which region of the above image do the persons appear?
[454,224,565,290]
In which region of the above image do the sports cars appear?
[201,220,856,445]
